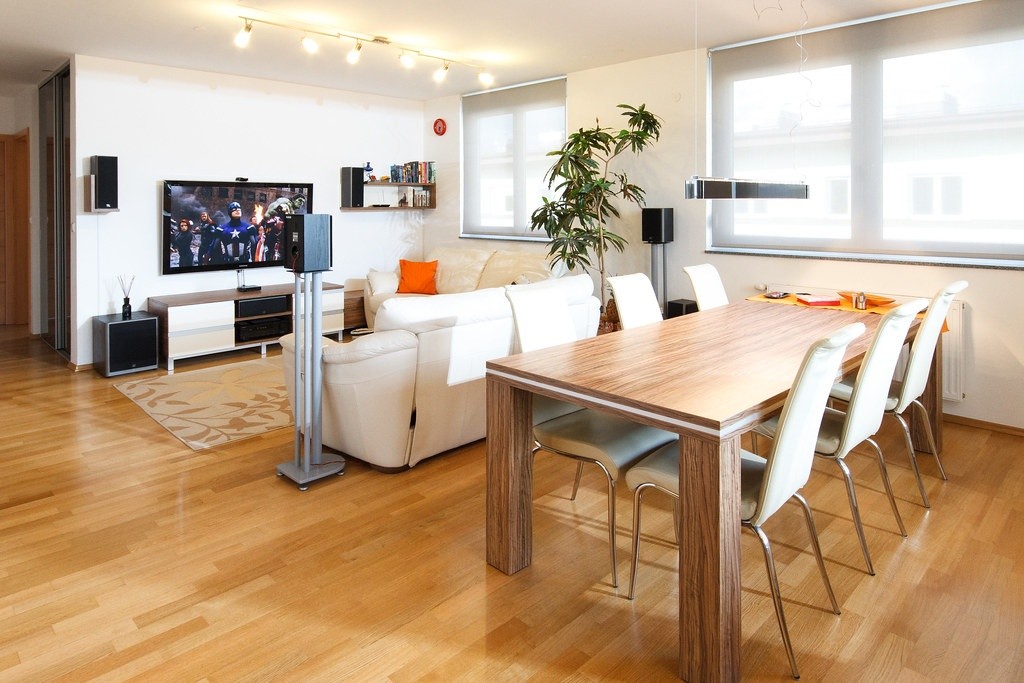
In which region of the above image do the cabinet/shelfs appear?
[149,282,345,373]
[364,181,437,210]
[93,309,158,378]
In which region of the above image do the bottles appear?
[363,162,373,183]
[857,292,865,310]
[122,298,131,320]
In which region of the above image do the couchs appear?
[276,248,600,475]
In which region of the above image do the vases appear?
[123,298,131,318]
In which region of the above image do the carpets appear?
[114,354,296,451]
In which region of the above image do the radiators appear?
[752,283,967,402]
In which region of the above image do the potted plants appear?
[526,102,665,336]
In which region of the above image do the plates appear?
[838,292,895,306]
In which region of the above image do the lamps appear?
[236,16,493,87]
[684,1,809,200]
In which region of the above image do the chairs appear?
[504,266,968,680]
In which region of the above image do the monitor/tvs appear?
[162,180,313,276]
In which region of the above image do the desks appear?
[485,293,944,682]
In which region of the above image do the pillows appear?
[395,259,439,294]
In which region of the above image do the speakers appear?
[341,167,365,208]
[91,311,160,378]
[236,295,286,317]
[284,214,332,272]
[641,208,674,242]
[90,156,118,209]
